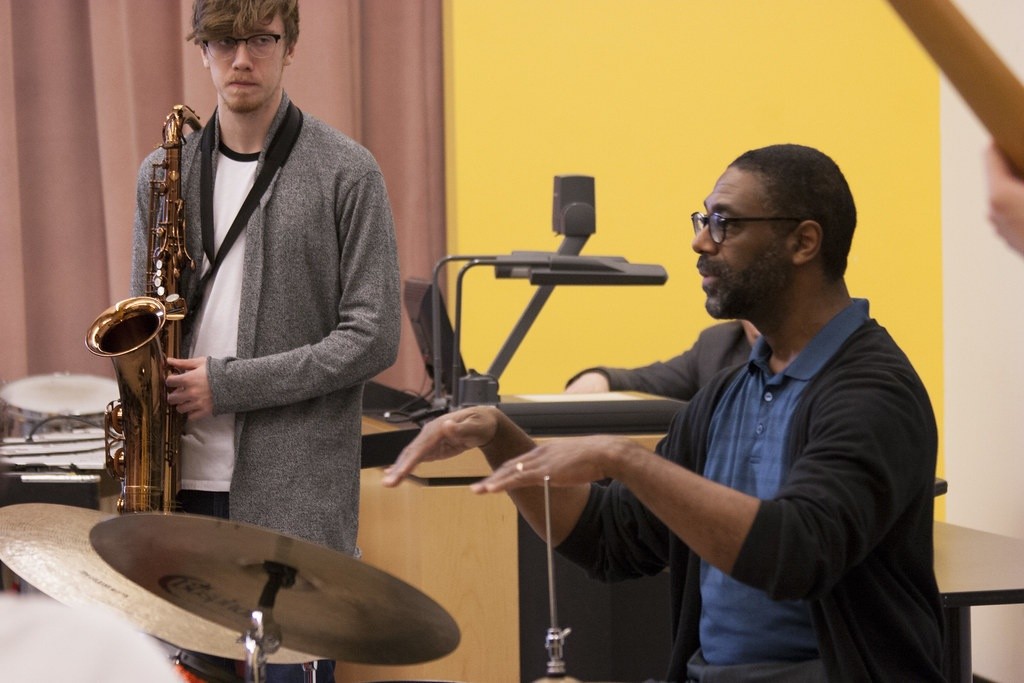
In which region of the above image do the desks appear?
[932,519,1024,683]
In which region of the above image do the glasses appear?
[691,212,826,245]
[201,34,287,60]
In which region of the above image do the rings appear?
[516,463,523,472]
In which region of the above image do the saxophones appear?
[77,99,209,526]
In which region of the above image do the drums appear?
[0,370,122,436]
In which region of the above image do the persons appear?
[562,319,762,402]
[381,143,951,683]
[131,1,401,683]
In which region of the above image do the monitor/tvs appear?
[403,278,467,397]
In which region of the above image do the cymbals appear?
[83,506,466,671]
[0,492,341,672]
